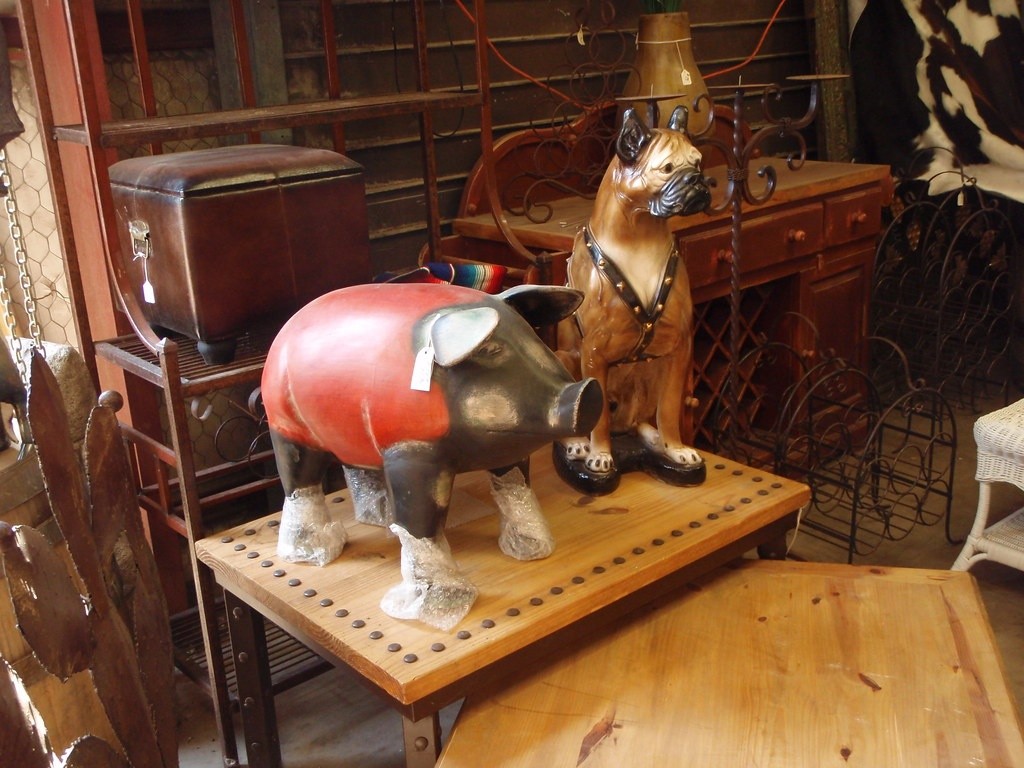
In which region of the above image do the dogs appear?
[539,104,714,472]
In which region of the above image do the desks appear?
[450,103,894,482]
[434,556,1024,768]
[194,436,810,768]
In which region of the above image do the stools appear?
[107,143,366,367]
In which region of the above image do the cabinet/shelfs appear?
[17,1,1024,768]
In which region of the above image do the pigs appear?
[261,282,606,613]
